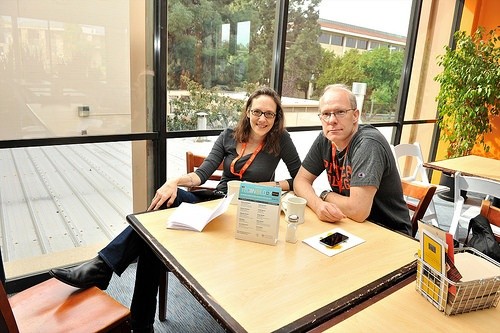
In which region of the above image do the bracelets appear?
[276,181,279,188]
[187,174,195,186]
[321,190,330,200]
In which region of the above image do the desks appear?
[422,152,500,197]
[124,189,499,332]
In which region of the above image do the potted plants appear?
[429,25,499,203]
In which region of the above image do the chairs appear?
[450,170,500,249]
[394,179,438,238]
[389,142,450,232]
[0,246,130,333]
[186,151,226,193]
[475,200,500,253]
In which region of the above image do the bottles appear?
[286,215,299,243]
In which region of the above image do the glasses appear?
[249,108,277,118]
[317,108,357,119]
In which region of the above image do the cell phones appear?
[319,232,349,248]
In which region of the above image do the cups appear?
[226,180,242,205]
[281,196,307,225]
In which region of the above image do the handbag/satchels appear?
[462,214,499,265]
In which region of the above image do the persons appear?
[292,84,412,238]
[47,86,303,333]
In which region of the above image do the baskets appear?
[413,246,500,316]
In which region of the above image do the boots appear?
[119,321,155,333]
[48,254,113,290]
